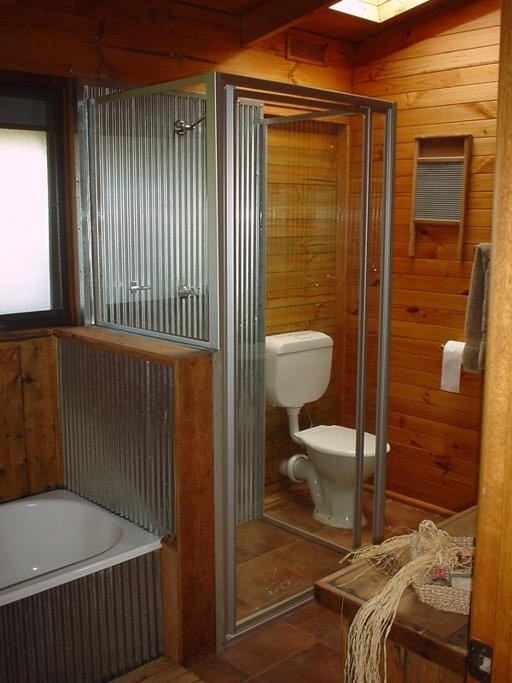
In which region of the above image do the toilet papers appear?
[440,340,467,394]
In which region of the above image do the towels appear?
[462,242,493,373]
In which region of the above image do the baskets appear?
[411,535,473,615]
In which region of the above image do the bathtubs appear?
[0,489,161,606]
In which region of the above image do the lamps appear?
[328,0,428,23]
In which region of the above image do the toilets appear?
[265,330,391,531]
